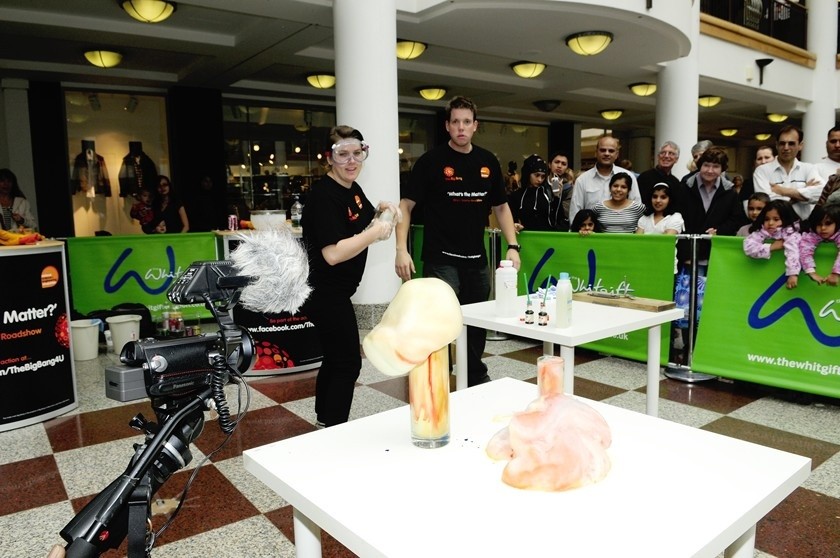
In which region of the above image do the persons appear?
[506,157,522,185]
[129,176,189,234]
[752,127,826,220]
[0,169,38,233]
[301,126,402,429]
[394,96,521,387]
[505,127,840,365]
[588,172,646,234]
[743,199,801,289]
[634,182,685,234]
[569,209,602,236]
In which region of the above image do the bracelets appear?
[507,245,522,252]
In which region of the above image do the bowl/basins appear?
[249,210,287,231]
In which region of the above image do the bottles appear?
[556,272,572,328]
[524,301,534,324]
[538,302,547,326]
[161,312,183,332]
[495,260,518,317]
[291,196,303,235]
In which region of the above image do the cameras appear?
[121,324,256,424]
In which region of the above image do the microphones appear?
[208,224,316,314]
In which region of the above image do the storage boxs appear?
[105,366,148,402]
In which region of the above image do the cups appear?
[104,330,113,346]
[70,319,101,360]
[537,356,565,395]
[22,228,32,235]
[408,344,449,450]
[106,315,142,355]
[551,178,560,192]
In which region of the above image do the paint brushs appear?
[524,273,530,304]
[543,274,551,303]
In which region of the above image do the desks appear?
[455,293,684,418]
[0,239,79,433]
[212,227,303,319]
[243,378,810,558]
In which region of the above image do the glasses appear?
[157,183,169,189]
[330,140,370,164]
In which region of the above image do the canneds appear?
[228,215,238,231]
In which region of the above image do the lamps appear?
[83,0,788,140]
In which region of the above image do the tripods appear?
[551,177,560,192]
